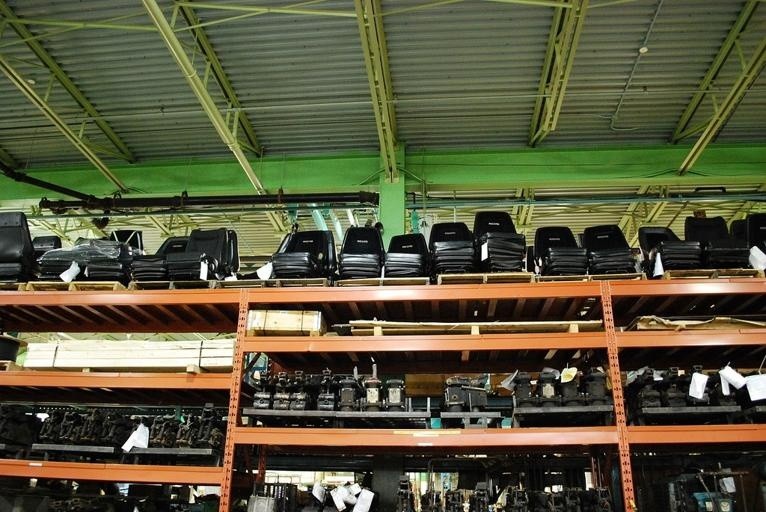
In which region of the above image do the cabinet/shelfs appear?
[607,278,766,511]
[225,280,631,510]
[0,289,243,511]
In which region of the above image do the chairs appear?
[1,210,765,279]
